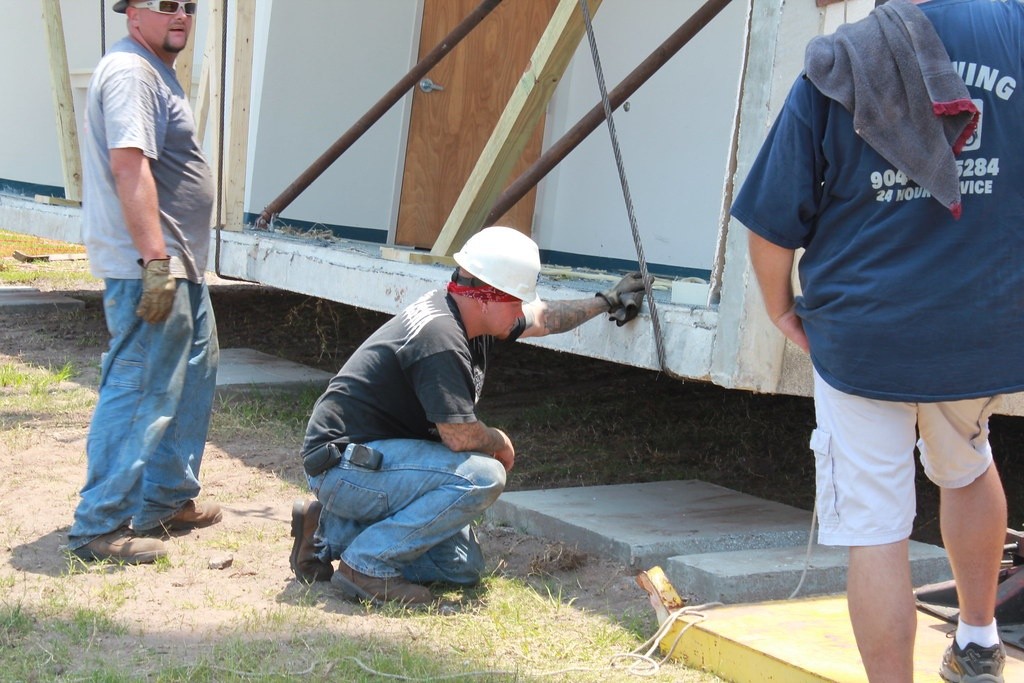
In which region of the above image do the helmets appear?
[452,225,543,305]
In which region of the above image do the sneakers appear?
[69,524,168,565]
[329,556,434,610]
[132,499,223,536]
[287,499,335,587]
[937,624,1007,683]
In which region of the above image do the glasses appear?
[129,0,198,16]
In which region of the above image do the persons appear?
[69,0,220,562]
[289,225,655,607]
[725,1,1022,681]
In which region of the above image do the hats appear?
[112,0,129,14]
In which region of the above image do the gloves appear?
[135,254,176,326]
[594,271,656,314]
[608,290,646,328]
